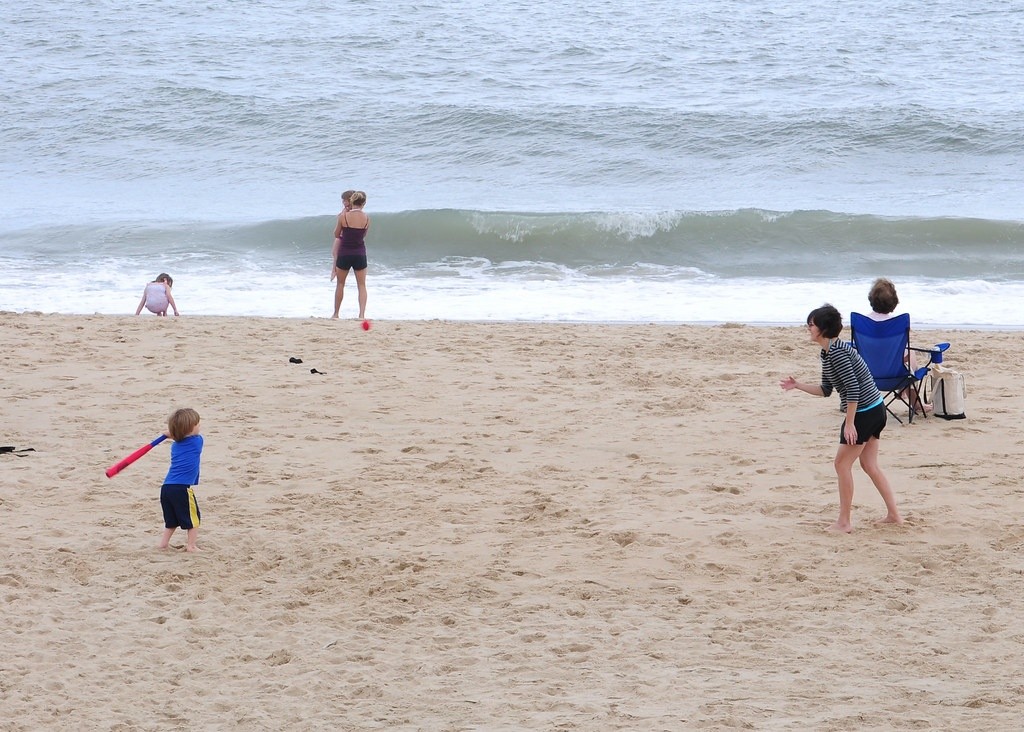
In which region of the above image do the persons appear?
[780,304,901,535]
[866,279,931,411]
[160,408,204,552]
[135,273,179,317]
[330,190,371,320]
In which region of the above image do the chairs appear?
[843,311,950,425]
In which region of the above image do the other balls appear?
[362,322,369,331]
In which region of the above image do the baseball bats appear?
[105,434,167,478]
